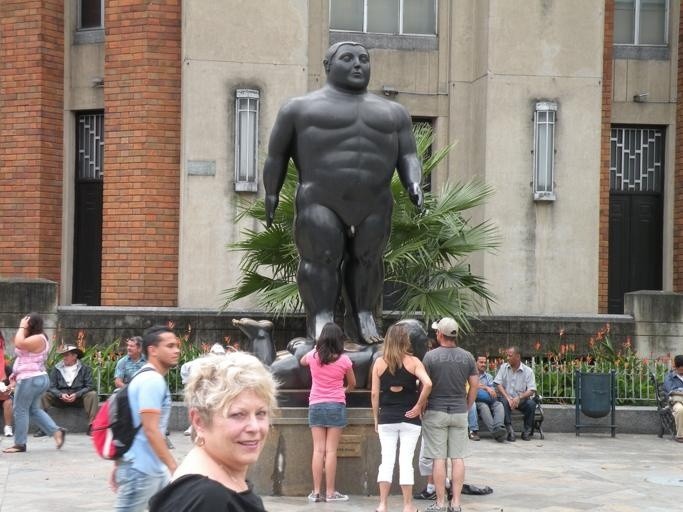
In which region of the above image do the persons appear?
[662,355,683,443]
[262,41,425,344]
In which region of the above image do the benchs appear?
[653,377,683,441]
[468,392,544,439]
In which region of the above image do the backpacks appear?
[94,367,158,459]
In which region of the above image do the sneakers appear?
[420,501,447,512]
[308,491,320,501]
[326,492,350,502]
[413,481,454,501]
[447,501,463,512]
[3,425,13,438]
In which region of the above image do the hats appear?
[56,344,85,359]
[432,317,460,337]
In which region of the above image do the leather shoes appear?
[470,425,534,443]
[32,426,66,450]
[4,444,25,453]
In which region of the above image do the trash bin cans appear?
[573,368,616,436]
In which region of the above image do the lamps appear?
[531,101,559,203]
[232,87,260,193]
